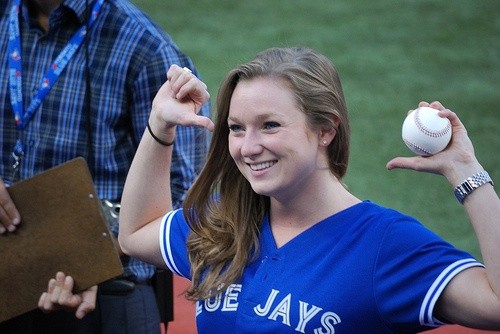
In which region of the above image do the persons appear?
[0,0,208,334]
[117,46,500,334]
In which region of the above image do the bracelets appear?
[146,120,176,146]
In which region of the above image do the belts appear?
[99,280,135,293]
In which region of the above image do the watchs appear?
[453,170,494,205]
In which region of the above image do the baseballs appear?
[401,105,452,158]
